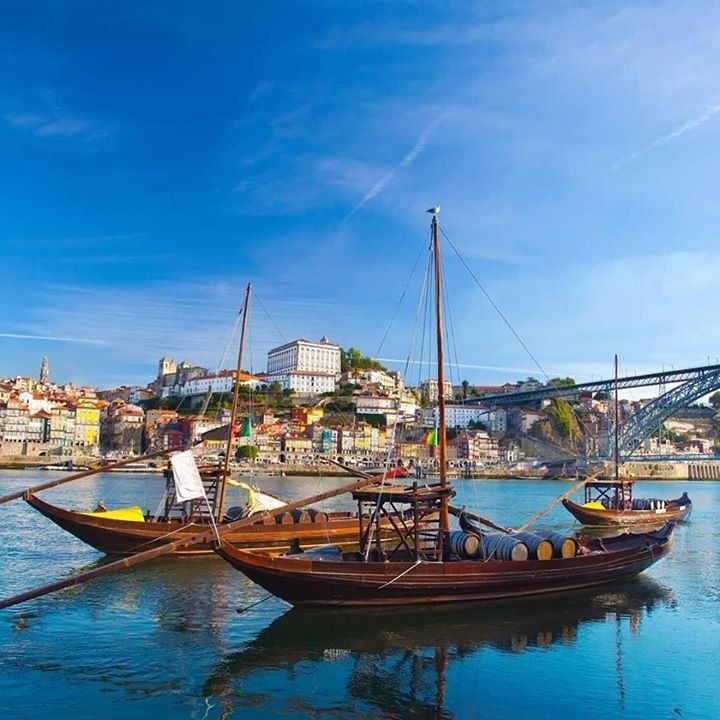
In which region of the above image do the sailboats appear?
[24,283,440,557]
[562,354,691,528]
[214,205,677,610]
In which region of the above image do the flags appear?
[233,419,250,437]
[201,424,231,441]
[422,426,437,447]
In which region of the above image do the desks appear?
[163,469,229,523]
[585,480,634,510]
[353,485,451,561]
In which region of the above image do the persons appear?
[92,500,106,513]
[618,491,623,509]
[144,510,152,522]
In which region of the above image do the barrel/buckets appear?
[434,530,481,558]
[481,535,528,561]
[227,506,328,525]
[515,533,554,559]
[534,531,578,558]
[632,499,666,510]
